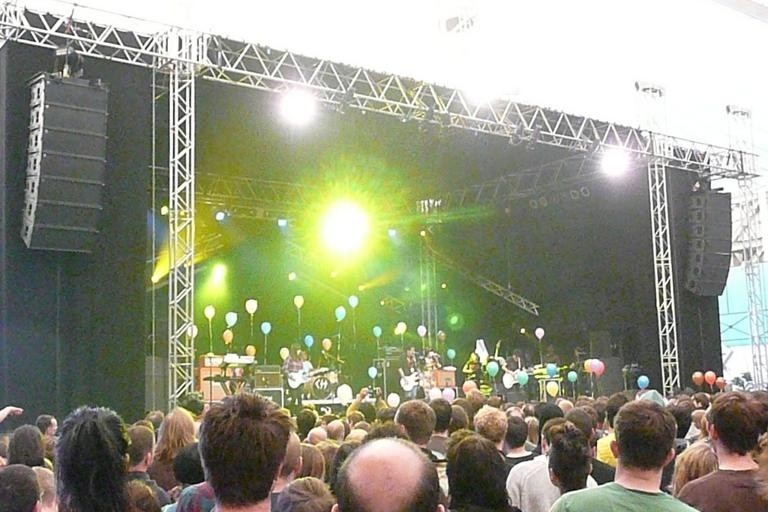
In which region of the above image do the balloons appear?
[368,367,378,379]
[247,345,256,357]
[638,375,650,390]
[335,384,456,404]
[546,358,605,396]
[692,371,727,389]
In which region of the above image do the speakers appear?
[601,356,625,395]
[683,192,732,298]
[19,71,111,255]
[589,330,611,359]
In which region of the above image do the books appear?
[335,306,347,322]
[223,329,233,344]
[438,330,446,345]
[374,326,384,338]
[260,321,271,334]
[535,328,545,341]
[305,335,312,348]
[203,305,213,318]
[348,294,360,309]
[397,323,406,334]
[244,299,260,315]
[321,337,331,351]
[418,325,426,336]
[294,295,303,308]
[225,311,237,328]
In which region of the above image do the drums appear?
[303,375,332,400]
[324,370,339,386]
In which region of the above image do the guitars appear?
[503,367,535,389]
[399,372,430,391]
[287,366,329,389]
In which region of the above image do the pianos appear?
[203,376,252,383]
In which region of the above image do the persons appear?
[399,346,441,401]
[462,348,528,402]
[280,342,312,410]
[1,387,767,511]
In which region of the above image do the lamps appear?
[335,87,542,152]
